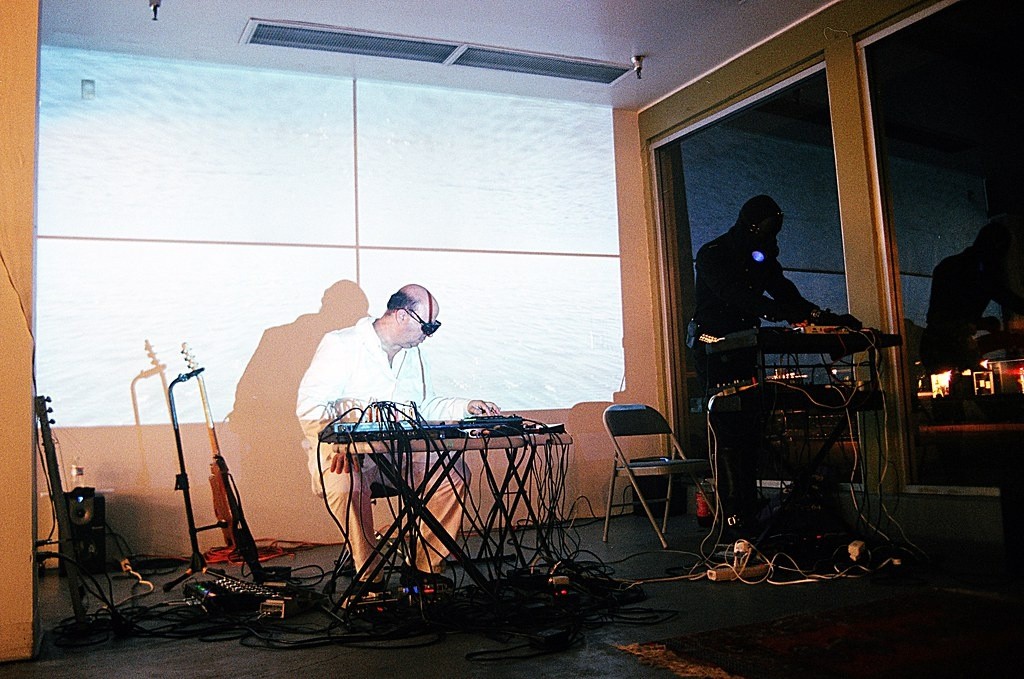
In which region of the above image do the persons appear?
[295,283,501,608]
[924,225,1022,353]
[694,195,863,499]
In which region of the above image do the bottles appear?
[696,474,715,529]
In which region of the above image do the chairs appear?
[603,404,717,549]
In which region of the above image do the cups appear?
[71,466,86,488]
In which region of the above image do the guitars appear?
[179,342,241,549]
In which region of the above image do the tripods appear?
[163,367,251,592]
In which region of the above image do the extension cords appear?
[707,566,769,581]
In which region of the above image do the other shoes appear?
[346,576,387,596]
[399,568,453,591]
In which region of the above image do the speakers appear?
[58,494,107,578]
[630,457,688,518]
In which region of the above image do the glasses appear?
[397,306,441,337]
